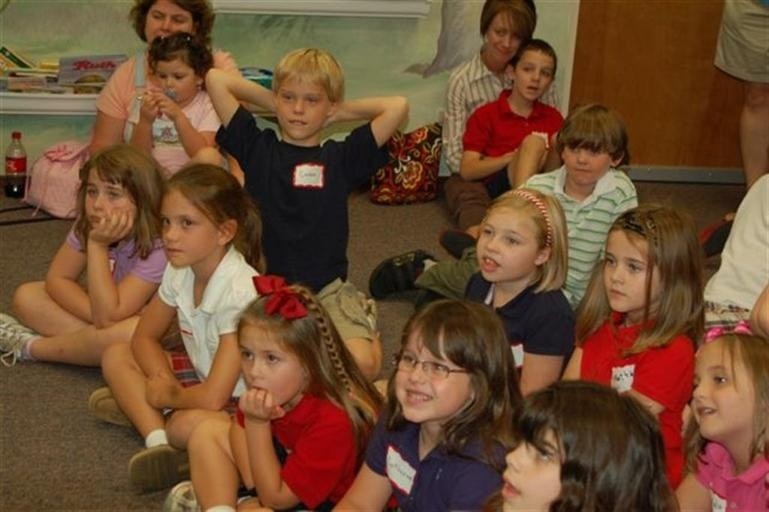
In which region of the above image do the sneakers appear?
[163,482,199,511]
[86,389,135,429]
[0,312,37,368]
[128,444,189,494]
[439,229,478,261]
[368,249,433,300]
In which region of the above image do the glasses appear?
[390,354,471,380]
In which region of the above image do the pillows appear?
[369,122,443,205]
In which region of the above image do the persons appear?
[502,381,685,512]
[514,103,642,303]
[558,203,705,491]
[86,165,297,493]
[703,169,769,337]
[674,321,767,510]
[709,1,769,196]
[440,0,560,239]
[123,33,229,178]
[460,40,566,187]
[332,297,521,512]
[448,188,575,398]
[205,45,409,385]
[167,278,384,512]
[88,0,246,161]
[1,143,178,366]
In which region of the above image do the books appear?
[0,44,128,94]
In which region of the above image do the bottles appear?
[5,132,28,200]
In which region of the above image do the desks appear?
[0,87,277,117]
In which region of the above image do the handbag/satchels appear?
[20,141,92,218]
